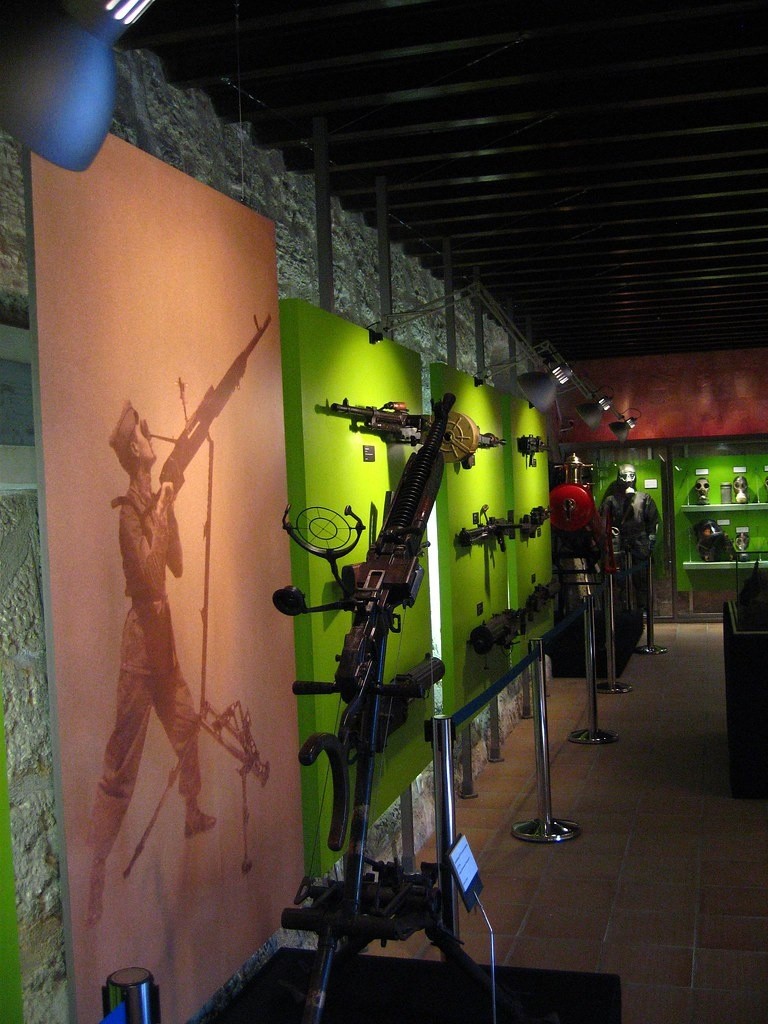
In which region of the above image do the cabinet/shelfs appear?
[671,442,768,614]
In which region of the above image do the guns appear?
[517,434,552,469]
[331,395,508,470]
[268,391,558,1024]
[458,503,524,553]
[525,579,562,621]
[519,505,551,539]
[468,605,535,655]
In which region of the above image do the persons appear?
[695,478,710,505]
[697,520,736,562]
[733,476,748,503]
[602,464,661,627]
[87,400,216,923]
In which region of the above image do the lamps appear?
[369,280,641,444]
[716,443,729,451]
[0,0,158,171]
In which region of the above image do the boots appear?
[84,858,107,926]
[183,802,218,841]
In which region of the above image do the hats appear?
[108,398,138,453]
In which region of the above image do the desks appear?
[724,600,768,799]
